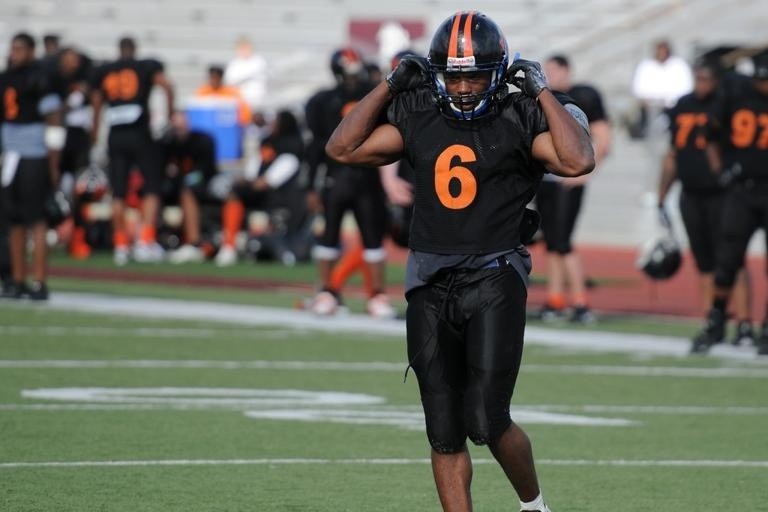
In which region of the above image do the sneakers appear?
[112,244,130,268]
[0,278,25,297]
[312,290,338,317]
[689,309,767,356]
[214,242,239,268]
[132,241,166,264]
[167,243,207,265]
[527,303,595,326]
[20,280,48,302]
[363,291,397,320]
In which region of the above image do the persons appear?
[0,33,419,318]
[324,10,598,512]
[536,35,768,357]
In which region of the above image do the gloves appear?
[384,54,435,97]
[502,59,550,101]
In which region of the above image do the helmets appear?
[693,45,768,84]
[330,49,366,78]
[390,51,417,71]
[636,239,682,281]
[428,9,511,90]
